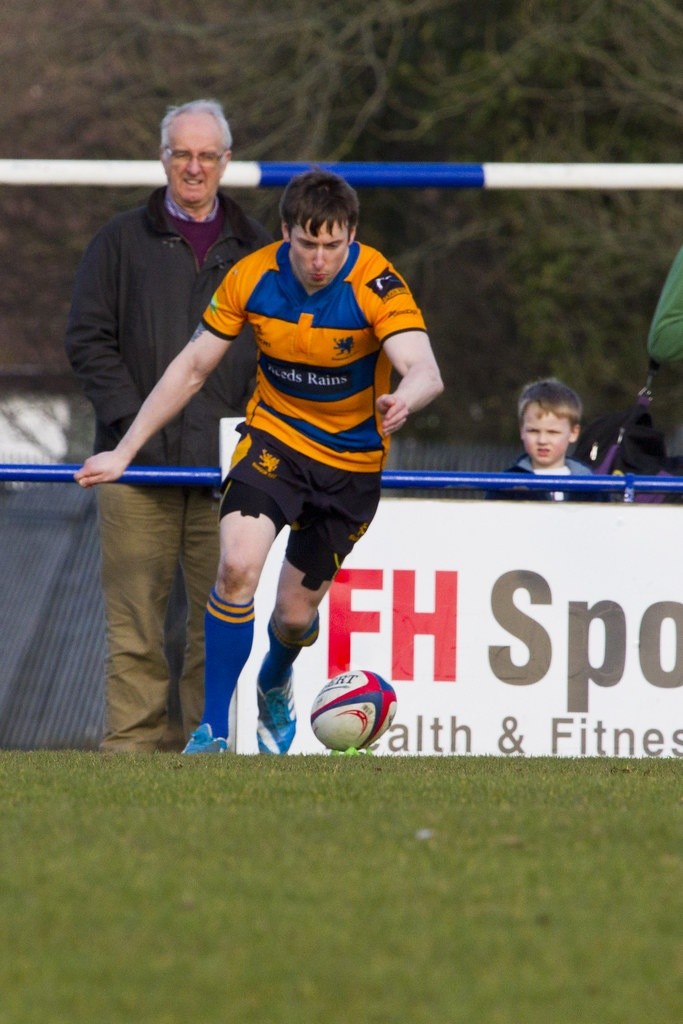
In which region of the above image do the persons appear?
[61,98,276,756]
[483,378,608,502]
[73,168,445,758]
[647,245,683,366]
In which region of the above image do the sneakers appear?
[257,652,296,758]
[180,723,228,755]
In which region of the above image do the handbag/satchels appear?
[575,408,683,503]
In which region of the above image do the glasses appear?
[165,148,227,166]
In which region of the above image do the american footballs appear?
[310,670,398,752]
